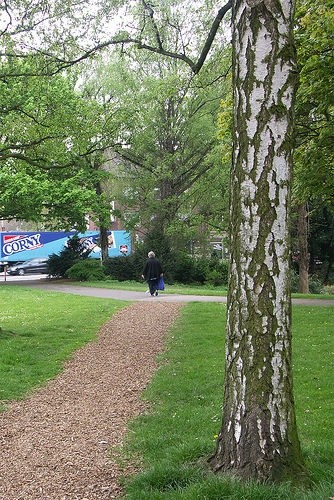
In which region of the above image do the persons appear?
[141,251,163,296]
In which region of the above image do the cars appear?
[6,258,48,276]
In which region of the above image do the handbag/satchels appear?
[157,276,164,291]
